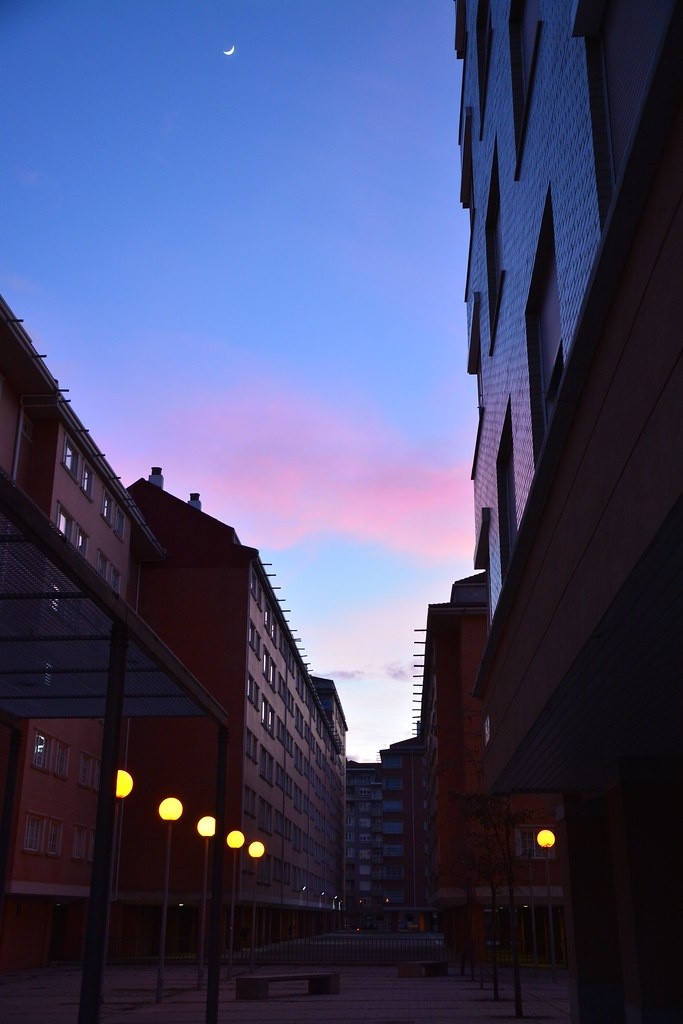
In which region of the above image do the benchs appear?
[235,971,341,1002]
[398,960,449,980]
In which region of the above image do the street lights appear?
[537,829,555,983]
[196,816,216,1000]
[226,830,246,980]
[156,796,183,1003]
[248,841,266,972]
[100,768,134,972]
